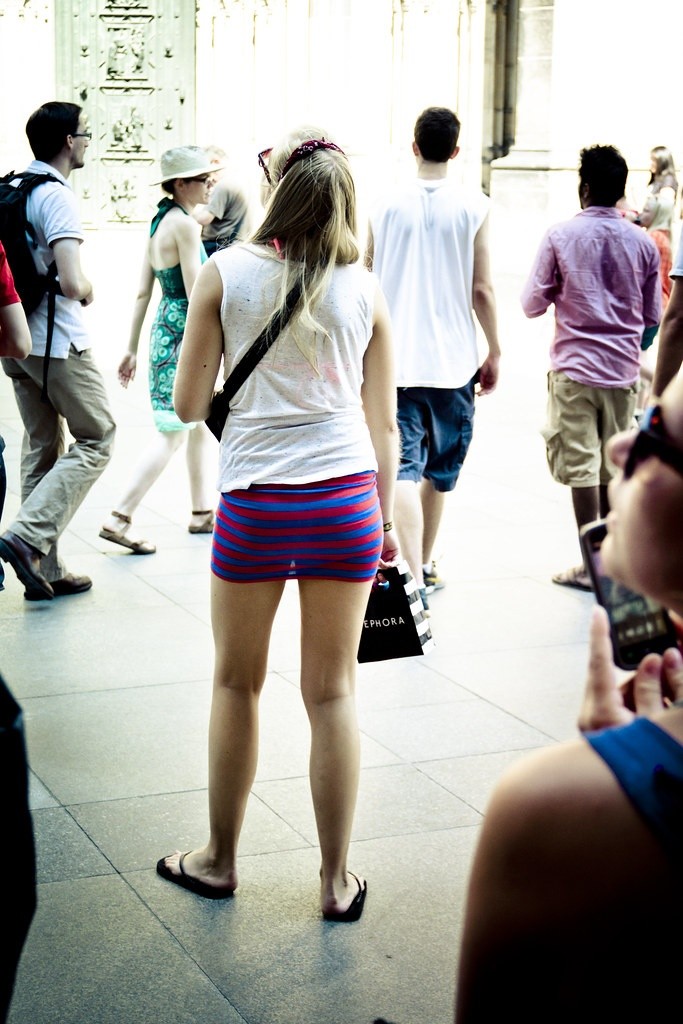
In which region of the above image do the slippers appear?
[156,849,234,899]
[322,870,368,922]
[552,567,594,595]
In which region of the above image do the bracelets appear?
[383,521,393,532]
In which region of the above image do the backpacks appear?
[0,170,66,318]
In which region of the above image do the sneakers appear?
[419,562,445,593]
[418,587,431,617]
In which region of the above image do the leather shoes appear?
[0,529,55,601]
[23,574,92,599]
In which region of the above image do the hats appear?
[149,145,224,186]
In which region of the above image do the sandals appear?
[188,510,215,536]
[98,511,156,554]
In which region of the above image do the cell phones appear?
[581,517,679,671]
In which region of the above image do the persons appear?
[455,362,683,1024]
[634,146,678,310]
[189,145,252,258]
[363,107,501,610]
[377,573,389,589]
[99,147,225,555]
[0,102,116,601]
[156,127,402,923]
[521,145,663,592]
[0,241,32,591]
[651,221,683,397]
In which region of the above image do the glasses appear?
[624,405,683,482]
[258,148,275,190]
[70,131,93,141]
[188,176,216,187]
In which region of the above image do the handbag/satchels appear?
[356,561,433,665]
[203,388,230,444]
